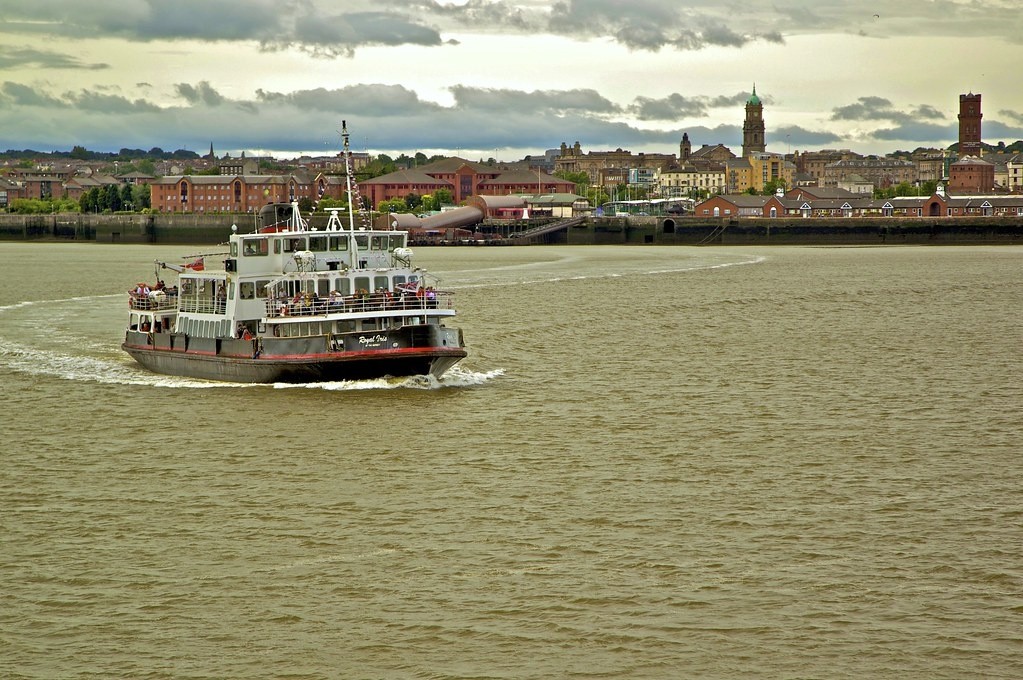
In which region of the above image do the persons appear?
[133,278,204,310]
[240,285,266,299]
[218,287,225,300]
[246,243,256,255]
[278,286,437,316]
[236,320,253,340]
[141,317,161,332]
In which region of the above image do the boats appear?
[121,121,468,383]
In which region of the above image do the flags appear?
[396,281,420,293]
[180,259,204,271]
